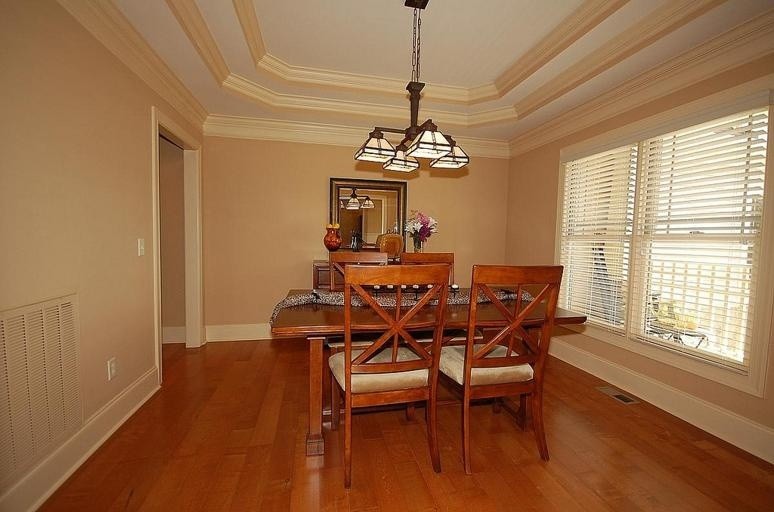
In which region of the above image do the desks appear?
[312,258,400,290]
[272,288,588,455]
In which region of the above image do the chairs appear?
[329,265,450,489]
[425,265,565,475]
[329,252,387,291]
[400,253,455,290]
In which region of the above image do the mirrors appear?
[330,179,407,252]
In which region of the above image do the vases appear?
[324,228,342,250]
[414,238,423,252]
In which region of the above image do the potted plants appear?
[349,228,359,250]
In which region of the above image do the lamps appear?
[339,187,375,210]
[354,0,468,172]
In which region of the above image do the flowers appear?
[326,222,340,229]
[406,212,437,240]
